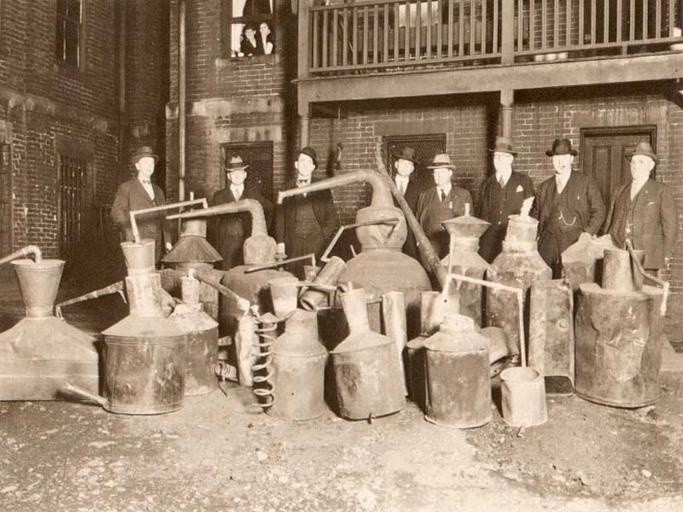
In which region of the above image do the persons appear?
[418,152,474,257]
[602,141,679,277]
[528,138,608,279]
[477,136,535,265]
[207,156,276,267]
[241,26,259,55]
[109,144,175,265]
[388,143,424,257]
[274,146,340,267]
[256,23,274,57]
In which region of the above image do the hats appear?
[390,146,420,162]
[427,153,457,168]
[489,143,517,156]
[546,138,577,156]
[132,146,160,164]
[625,142,659,165]
[289,147,318,170]
[224,152,250,172]
